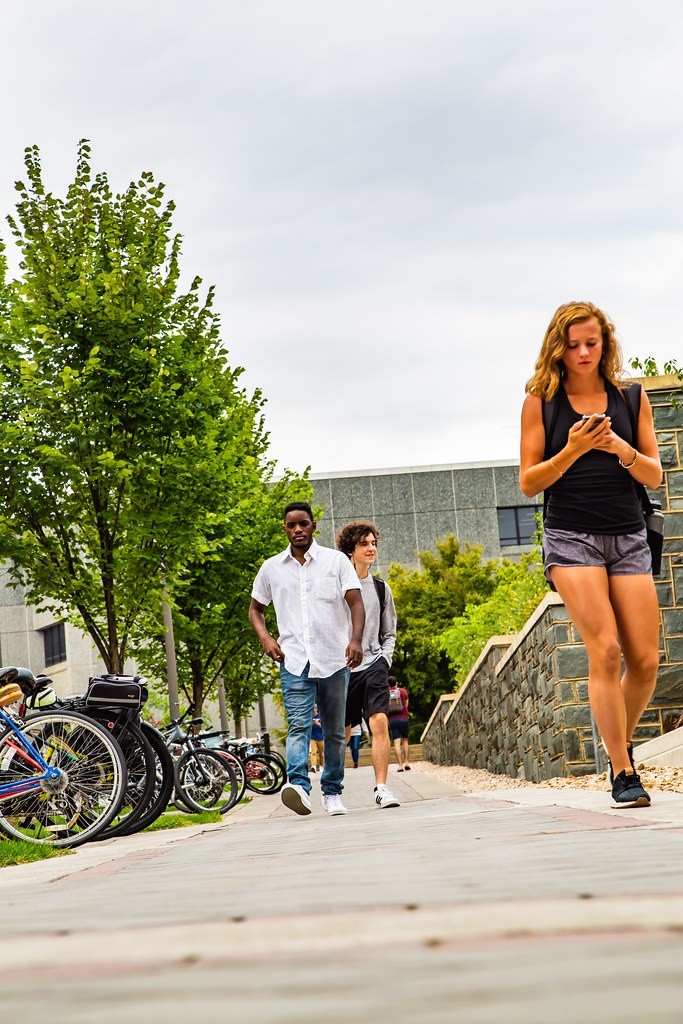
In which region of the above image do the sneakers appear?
[372,786,400,808]
[279,783,312,815]
[321,792,348,816]
[606,741,653,809]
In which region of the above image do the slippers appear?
[405,766,410,771]
[397,769,404,772]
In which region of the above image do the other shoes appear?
[352,763,358,768]
[310,766,317,774]
[319,765,324,773]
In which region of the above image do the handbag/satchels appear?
[82,672,150,712]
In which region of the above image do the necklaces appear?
[361,571,369,579]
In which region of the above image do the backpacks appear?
[388,688,403,712]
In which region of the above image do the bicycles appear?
[0,667,288,850]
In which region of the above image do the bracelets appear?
[619,449,638,469]
[550,458,563,477]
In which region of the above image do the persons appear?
[250,502,364,817]
[350,716,369,769]
[520,300,663,806]
[388,677,411,772]
[335,520,398,809]
[310,704,324,773]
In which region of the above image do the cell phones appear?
[583,414,606,431]
